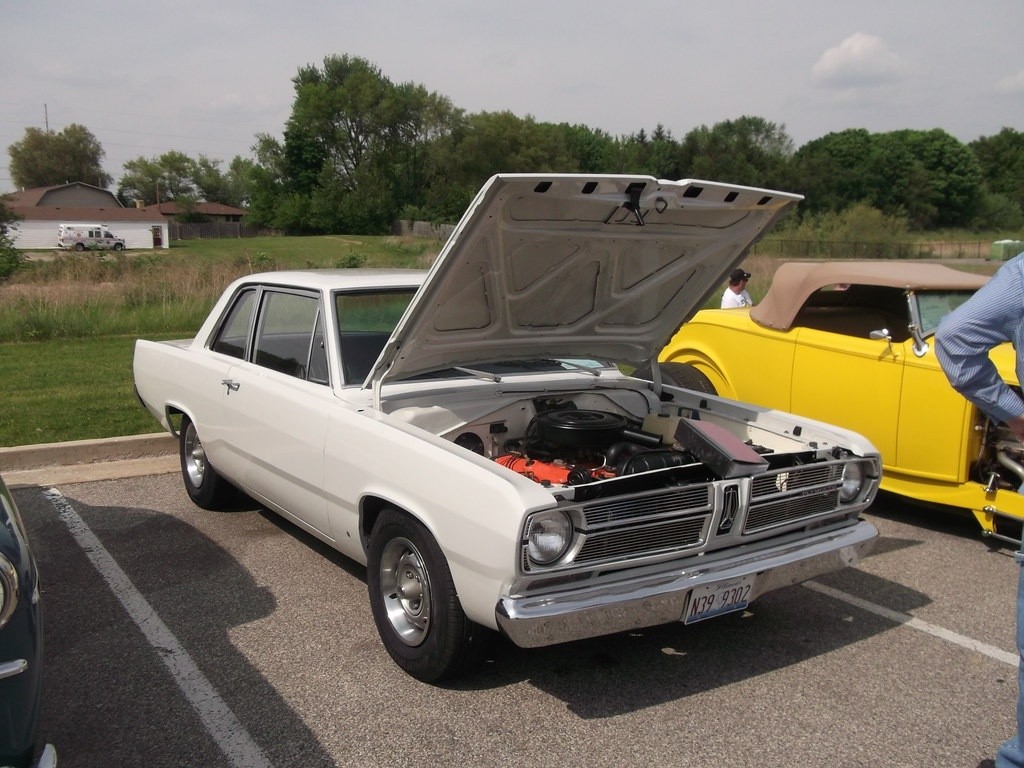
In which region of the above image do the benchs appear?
[792,306,907,343]
[215,332,391,386]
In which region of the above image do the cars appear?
[0,479,57,768]
[629,259,1023,565]
[132,173,882,682]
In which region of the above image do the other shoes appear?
[976,759,995,768]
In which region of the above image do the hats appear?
[730,268,751,279]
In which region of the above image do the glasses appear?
[744,278,748,282]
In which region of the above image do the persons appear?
[934,251,1024,768]
[721,269,753,309]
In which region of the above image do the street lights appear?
[157,181,161,210]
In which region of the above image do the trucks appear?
[57,224,126,251]
